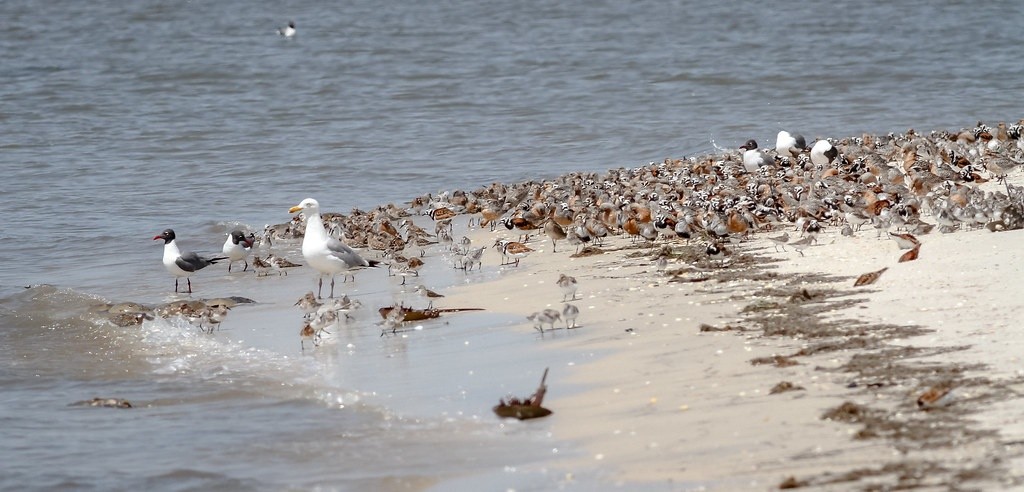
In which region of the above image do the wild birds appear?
[682,120,1024,288]
[918,381,966,408]
[377,274,580,338]
[365,156,703,285]
[276,21,297,37]
[92,396,133,408]
[152,198,389,347]
[739,132,839,171]
